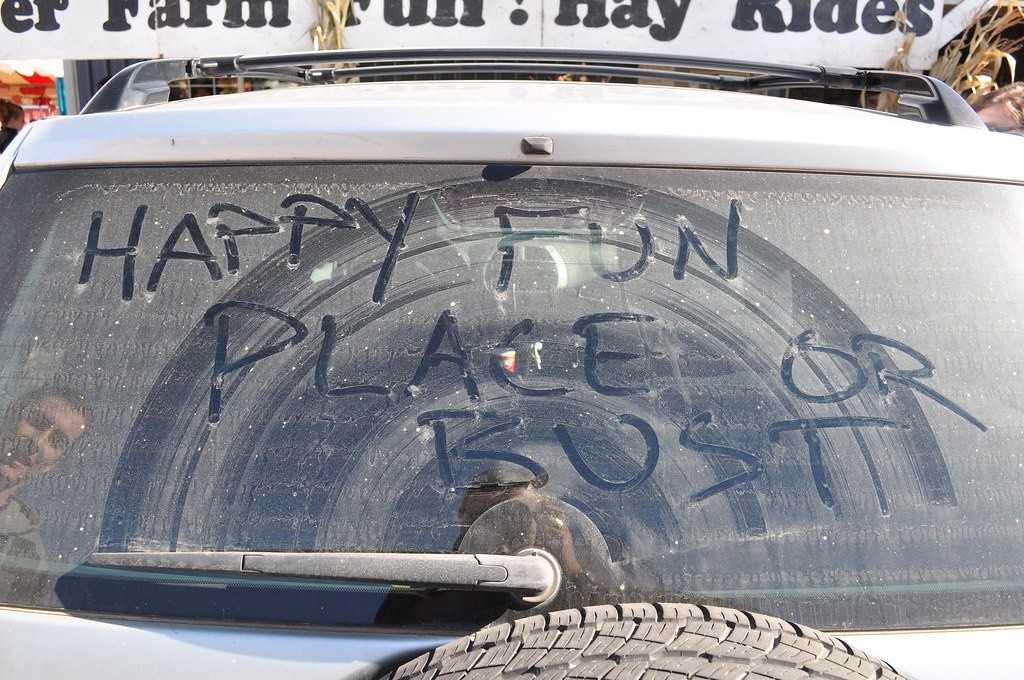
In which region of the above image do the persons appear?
[1,386,93,605]
[372,463,614,634]
[0,98,25,155]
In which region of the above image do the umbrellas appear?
[0,59,57,105]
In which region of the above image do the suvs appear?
[0,48,1023,680]
